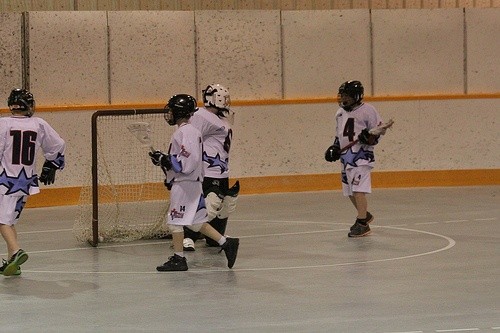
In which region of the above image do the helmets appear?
[202,84,232,108]
[7,89,36,118]
[337,80,365,112]
[163,94,199,126]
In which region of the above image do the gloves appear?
[149,151,168,166]
[325,145,339,162]
[39,160,60,185]
[358,129,375,145]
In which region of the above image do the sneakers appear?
[348,211,374,237]
[156,253,188,271]
[0,249,29,276]
[206,235,232,247]
[221,238,239,269]
[183,237,195,250]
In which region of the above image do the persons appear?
[149,94,240,272]
[0,87,66,276]
[183,83,234,251]
[323,80,385,238]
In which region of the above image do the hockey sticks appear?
[125,122,167,177]
[338,118,395,154]
